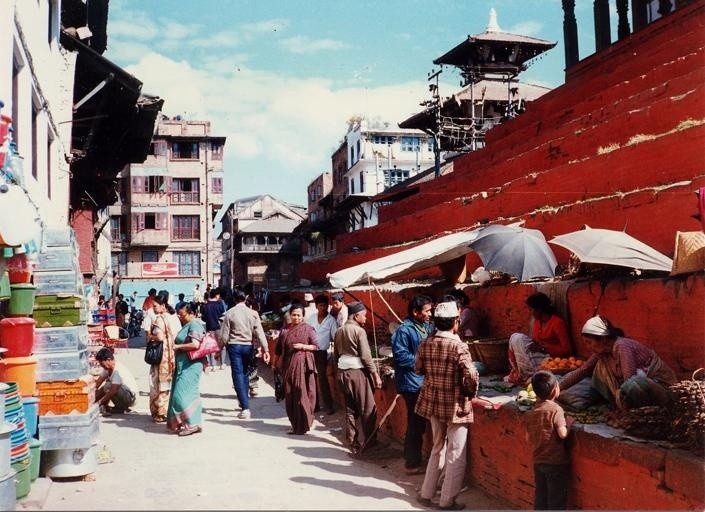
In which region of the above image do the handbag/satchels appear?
[275,381,285,402]
[185,332,220,361]
[145,339,163,365]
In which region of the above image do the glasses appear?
[456,319,461,324]
[178,313,187,319]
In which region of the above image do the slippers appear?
[404,467,426,476]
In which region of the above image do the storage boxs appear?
[33,225,101,452]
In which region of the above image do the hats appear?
[435,301,459,317]
[305,293,314,301]
[347,302,364,314]
[332,293,343,300]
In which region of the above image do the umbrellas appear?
[466,223,558,282]
[544,227,674,276]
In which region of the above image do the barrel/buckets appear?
[8,283,37,316]
[0,317,36,358]
[0,358,43,512]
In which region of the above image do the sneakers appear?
[250,388,258,396]
[179,426,202,436]
[417,493,432,507]
[439,501,465,510]
[239,409,250,419]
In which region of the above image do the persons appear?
[524,371,575,511]
[271,292,391,459]
[558,315,679,414]
[194,285,270,420]
[95,289,207,436]
[502,292,574,387]
[391,287,483,511]
